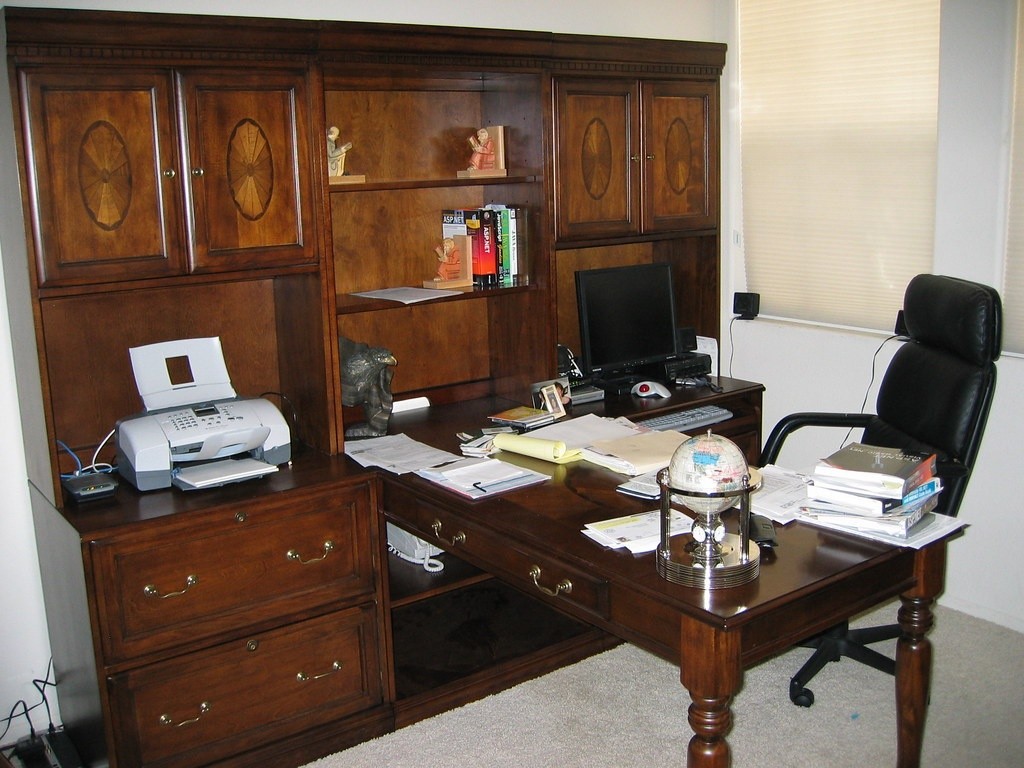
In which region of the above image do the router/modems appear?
[60,472,119,503]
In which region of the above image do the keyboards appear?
[638,404,733,432]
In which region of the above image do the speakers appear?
[733,292,760,320]
[676,327,697,353]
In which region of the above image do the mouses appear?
[630,381,672,399]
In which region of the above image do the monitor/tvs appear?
[575,263,680,398]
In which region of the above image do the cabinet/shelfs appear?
[0,6,764,768]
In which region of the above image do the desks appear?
[383,445,965,768]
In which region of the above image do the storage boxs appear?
[441,208,498,285]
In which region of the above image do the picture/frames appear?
[541,384,567,419]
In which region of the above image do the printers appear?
[114,337,291,492]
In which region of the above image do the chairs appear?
[754,271,1003,706]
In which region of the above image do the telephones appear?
[386,522,445,559]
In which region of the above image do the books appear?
[488,407,692,501]
[467,136,478,148]
[796,441,943,538]
[341,142,352,150]
[439,207,518,287]
[435,247,443,257]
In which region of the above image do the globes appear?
[654,430,762,591]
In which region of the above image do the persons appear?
[537,383,571,410]
[466,128,494,170]
[434,238,460,281]
[327,126,346,176]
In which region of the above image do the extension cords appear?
[14,734,44,760]
